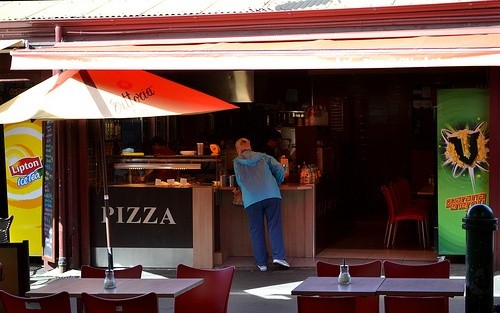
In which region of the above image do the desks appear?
[291,276,464,299]
[25,277,204,298]
[220,182,316,259]
[416,184,434,196]
[274,125,325,161]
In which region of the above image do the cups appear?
[229,175,235,187]
[167,179,175,184]
[180,178,187,184]
[429,176,434,184]
[276,112,303,125]
[220,175,227,187]
[197,143,203,155]
[156,179,161,185]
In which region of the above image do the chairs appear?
[316,260,382,313]
[81,264,142,278]
[78,293,159,313]
[380,179,430,249]
[296,296,357,313]
[0,290,71,313]
[174,263,235,313]
[383,260,450,280]
[384,296,449,313]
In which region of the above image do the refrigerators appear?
[433,86,489,259]
[0,117,43,257]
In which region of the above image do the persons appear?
[234,130,291,268]
[144,136,178,182]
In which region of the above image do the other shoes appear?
[272,259,291,268]
[258,264,268,271]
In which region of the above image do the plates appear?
[180,151,196,155]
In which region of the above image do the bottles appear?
[338,264,351,285]
[305,105,329,126]
[103,268,116,289]
[298,161,320,184]
[212,181,220,187]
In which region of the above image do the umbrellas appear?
[0,70,242,280]
